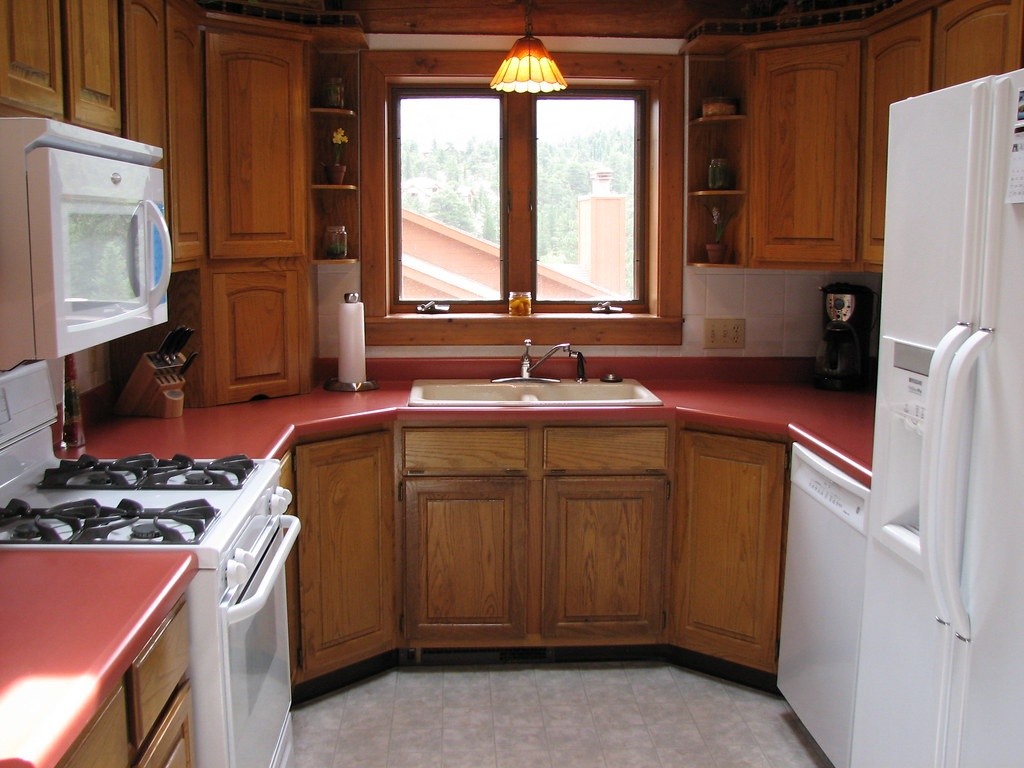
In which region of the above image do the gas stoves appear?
[0,359,282,569]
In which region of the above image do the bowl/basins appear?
[700,94,737,115]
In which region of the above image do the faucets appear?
[520,337,571,379]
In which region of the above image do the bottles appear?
[323,225,347,259]
[508,291,532,316]
[320,77,345,108]
[709,158,730,190]
[337,292,366,383]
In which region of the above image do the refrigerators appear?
[872,67,1024,768]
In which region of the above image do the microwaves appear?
[0,117,174,371]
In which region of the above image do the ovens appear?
[185,514,302,768]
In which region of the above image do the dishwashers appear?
[776,442,872,768]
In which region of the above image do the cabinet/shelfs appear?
[56,584,196,768]
[280,424,792,713]
[0,0,372,406]
[683,0,1024,268]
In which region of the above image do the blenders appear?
[817,282,881,392]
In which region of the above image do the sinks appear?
[420,384,529,403]
[529,383,647,401]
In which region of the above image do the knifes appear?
[154,324,199,377]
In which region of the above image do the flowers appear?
[710,205,728,244]
[329,126,349,166]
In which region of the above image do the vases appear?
[707,243,727,264]
[324,164,348,185]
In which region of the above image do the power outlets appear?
[703,318,747,350]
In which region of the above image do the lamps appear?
[489,0,567,93]
